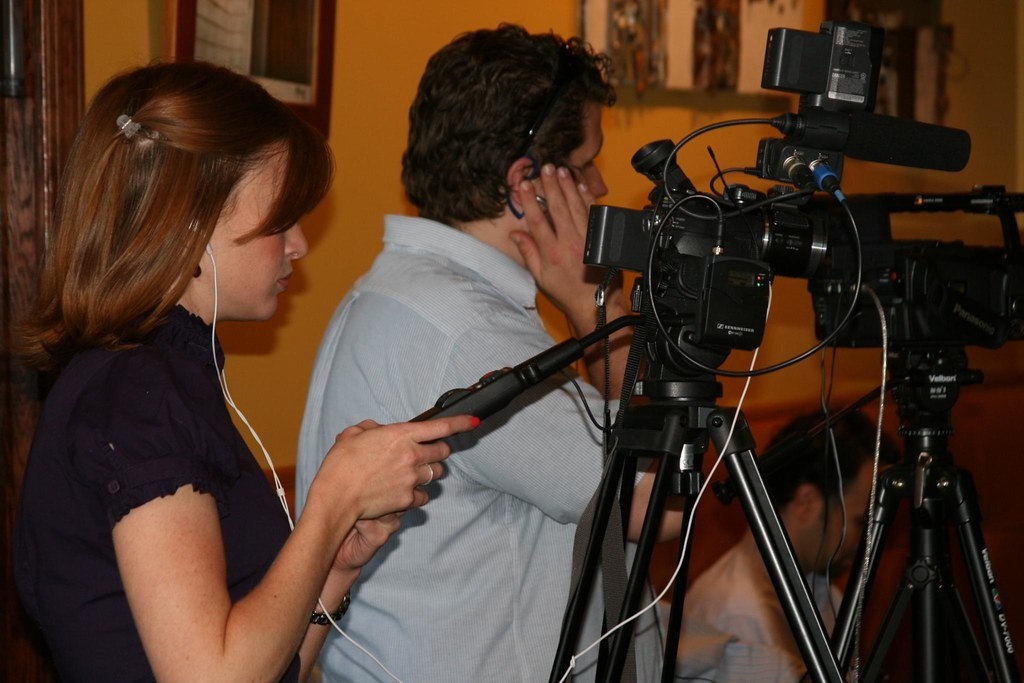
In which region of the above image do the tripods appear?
[395,284,1021,682]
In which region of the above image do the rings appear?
[420,463,434,486]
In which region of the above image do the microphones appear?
[775,106,973,173]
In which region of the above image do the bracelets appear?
[308,590,351,625]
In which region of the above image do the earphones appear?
[188,218,212,255]
[534,194,546,206]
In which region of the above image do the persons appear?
[672,404,898,683]
[292,22,687,683]
[12,60,482,683]
